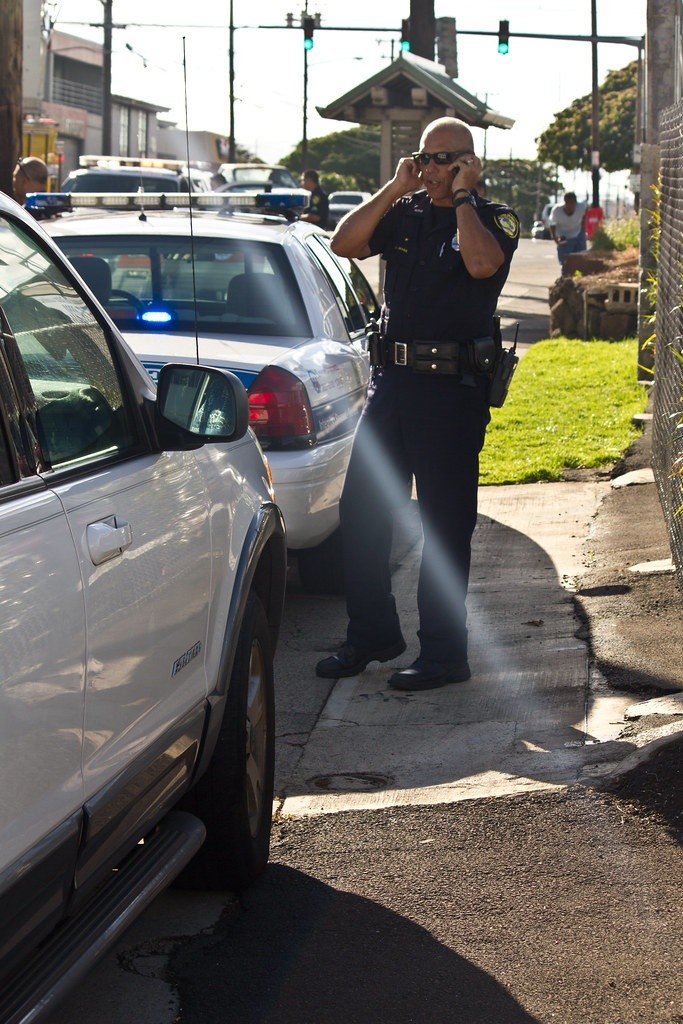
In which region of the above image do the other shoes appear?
[315,636,407,680]
[389,655,470,690]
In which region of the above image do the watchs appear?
[454,195,477,212]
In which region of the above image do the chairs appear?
[67,251,293,321]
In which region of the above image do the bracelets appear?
[452,188,471,203]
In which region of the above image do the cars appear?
[54,154,371,229]
[38,202,400,595]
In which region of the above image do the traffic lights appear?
[400,20,410,53]
[304,19,314,51]
[499,20,509,54]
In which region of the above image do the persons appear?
[316,117,518,691]
[549,192,587,278]
[12,156,48,206]
[210,173,226,193]
[294,170,329,229]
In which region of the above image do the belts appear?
[373,335,505,376]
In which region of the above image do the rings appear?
[466,159,473,164]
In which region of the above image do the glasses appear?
[412,150,473,165]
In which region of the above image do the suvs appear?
[0,193,289,1024]
